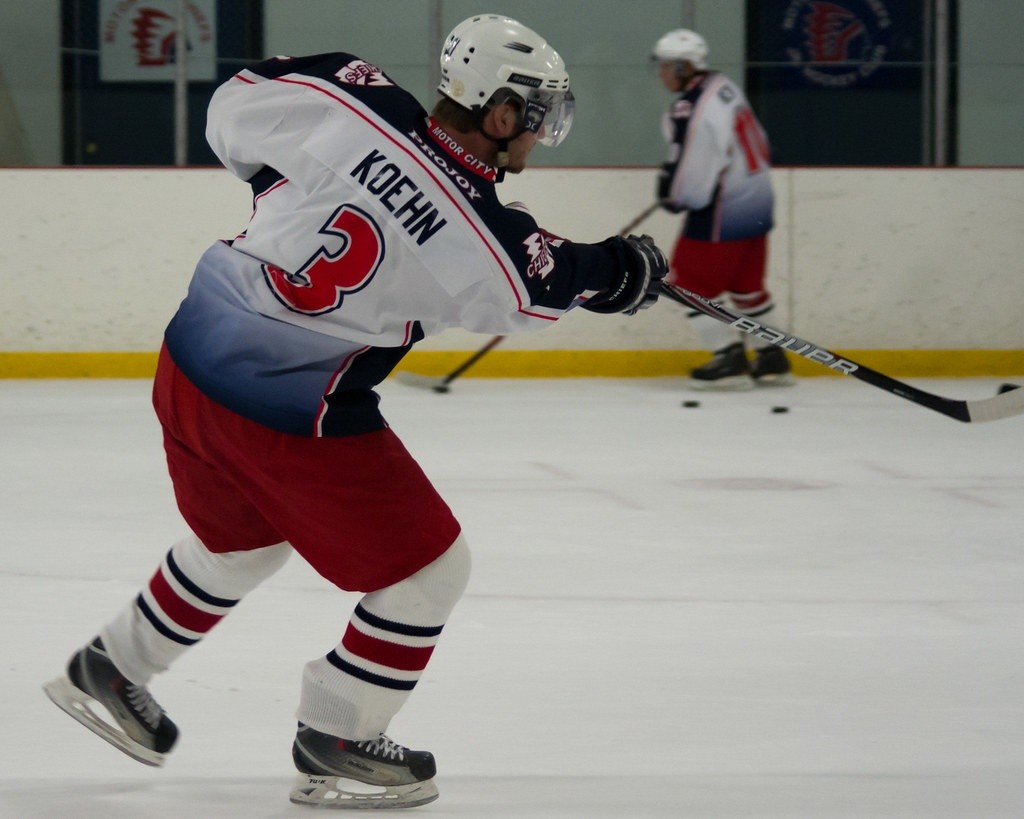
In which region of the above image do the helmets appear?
[651,28,710,71]
[437,14,568,110]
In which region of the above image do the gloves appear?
[624,234,669,316]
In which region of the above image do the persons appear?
[39,10,673,810]
[643,24,808,408]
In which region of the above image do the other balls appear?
[681,402,698,407]
[770,407,788,414]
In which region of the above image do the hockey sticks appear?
[662,281,1024,424]
[395,199,661,394]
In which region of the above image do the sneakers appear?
[288,721,441,809]
[747,346,796,388]
[41,635,179,769]
[687,347,754,392]
